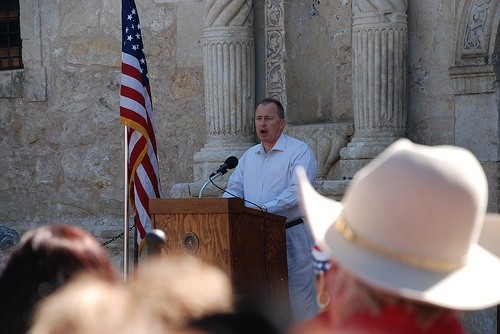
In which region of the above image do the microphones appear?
[210,156,238,177]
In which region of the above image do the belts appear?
[285,216,304,229]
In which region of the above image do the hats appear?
[296,139,500,310]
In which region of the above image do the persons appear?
[0,136,500,334]
[221,99,323,324]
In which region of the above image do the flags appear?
[120,0,163,256]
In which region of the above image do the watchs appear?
[261,205,267,212]
